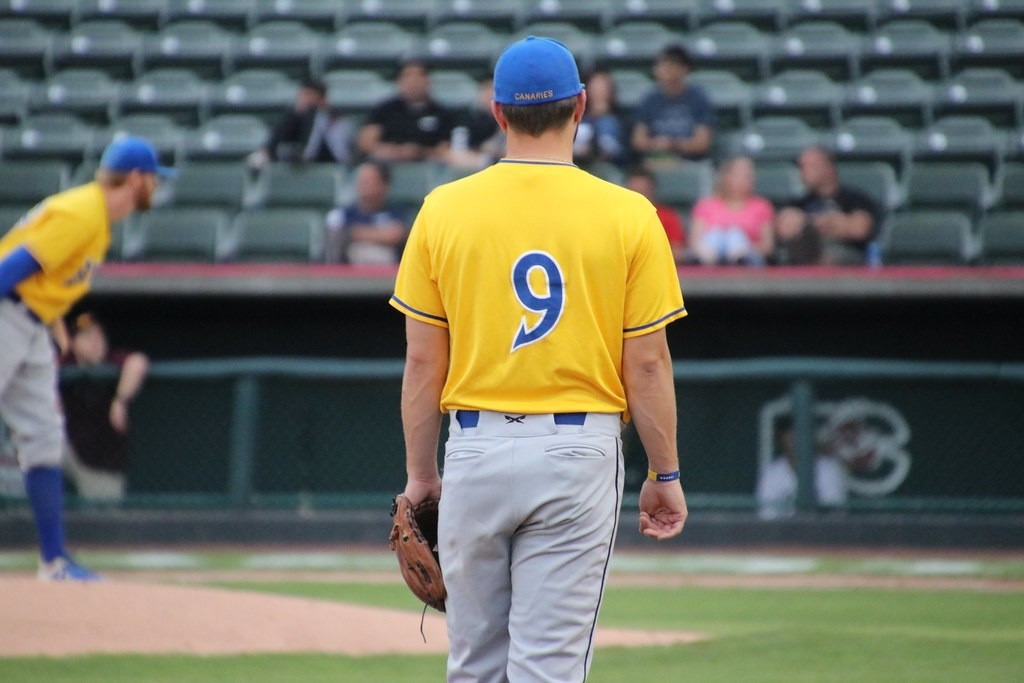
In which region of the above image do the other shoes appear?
[36,555,103,582]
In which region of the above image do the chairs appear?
[0,0,1024,279]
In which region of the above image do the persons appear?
[690,155,779,269]
[247,74,343,184]
[625,167,692,265]
[59,308,151,470]
[388,33,688,683]
[343,156,406,267]
[0,134,177,579]
[773,147,890,270]
[630,41,714,201]
[354,56,455,215]
[451,64,629,186]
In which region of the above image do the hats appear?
[493,35,585,105]
[100,137,181,176]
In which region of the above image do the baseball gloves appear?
[390,495,448,617]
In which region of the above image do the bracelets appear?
[115,395,128,406]
[647,469,681,482]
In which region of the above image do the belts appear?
[456,410,587,428]
[7,290,40,323]
